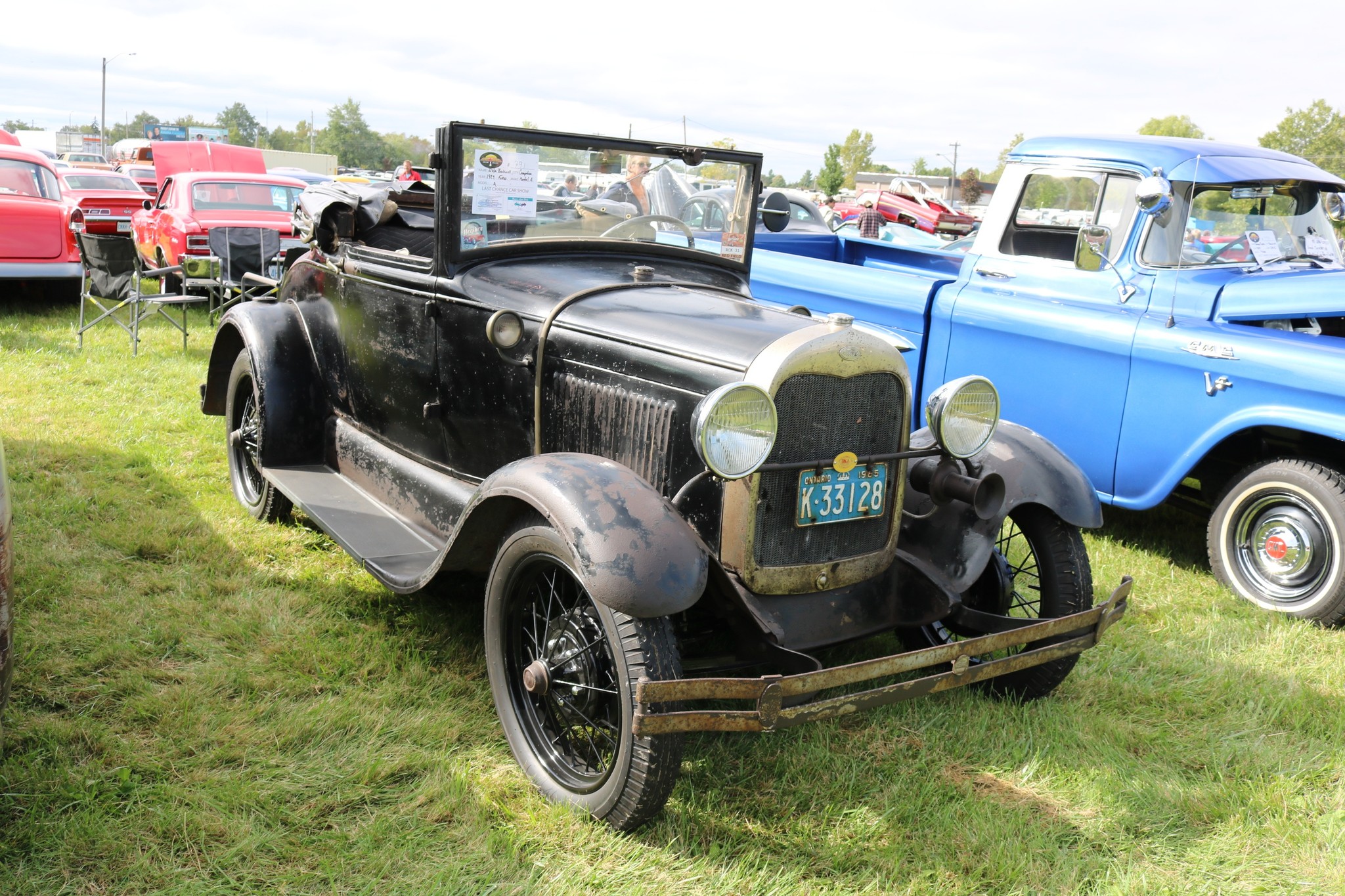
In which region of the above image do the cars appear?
[198,123,1134,838]
[1,127,1249,301]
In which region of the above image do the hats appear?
[865,200,872,208]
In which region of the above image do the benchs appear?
[194,202,283,212]
[365,218,565,259]
[1000,229,1079,262]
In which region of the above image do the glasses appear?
[638,162,652,168]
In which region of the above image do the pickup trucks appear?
[656,133,1345,628]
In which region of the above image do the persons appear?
[856,200,887,239]
[554,155,653,225]
[191,133,228,144]
[397,160,423,181]
[146,127,163,140]
[819,197,836,231]
[1182,229,1212,254]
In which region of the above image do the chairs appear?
[73,231,208,357]
[241,248,312,302]
[208,226,279,325]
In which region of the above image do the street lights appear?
[936,154,955,207]
